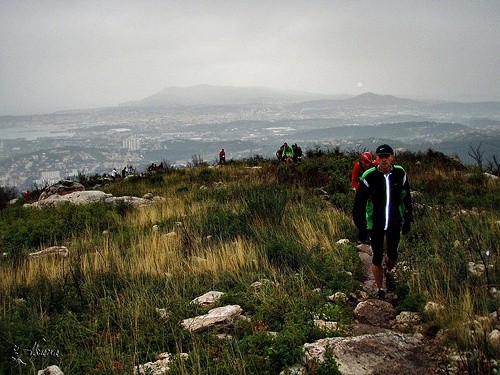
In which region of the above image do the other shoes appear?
[378,289,386,301]
[384,268,396,292]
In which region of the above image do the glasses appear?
[377,154,392,158]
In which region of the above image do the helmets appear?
[361,152,372,163]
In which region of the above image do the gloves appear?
[358,230,371,245]
[402,221,411,235]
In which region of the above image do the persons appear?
[219,149,225,162]
[19,190,29,199]
[111,166,135,182]
[352,152,374,240]
[352,144,413,299]
[147,162,164,171]
[276,142,302,164]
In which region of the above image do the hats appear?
[376,144,393,155]
